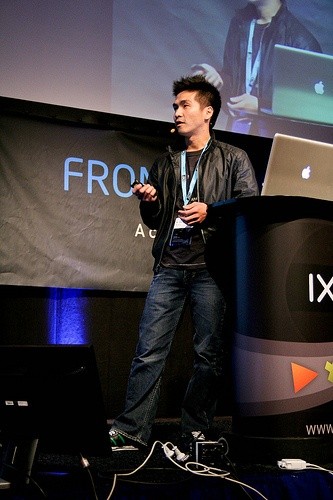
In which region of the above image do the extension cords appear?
[162,453,194,467]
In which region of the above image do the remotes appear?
[130,180,144,188]
[190,64,208,74]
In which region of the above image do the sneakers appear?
[109,429,140,452]
[181,430,208,459]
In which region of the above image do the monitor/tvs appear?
[0,345,112,458]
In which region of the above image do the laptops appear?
[261,133,333,201]
[260,44,333,125]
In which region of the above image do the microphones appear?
[170,128,176,133]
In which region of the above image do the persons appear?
[108,74,260,452]
[191,0,323,138]
